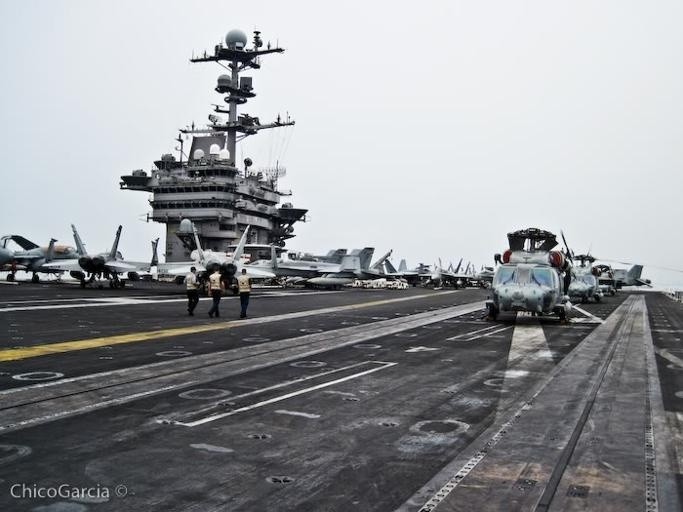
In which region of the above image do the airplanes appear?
[1,220,652,325]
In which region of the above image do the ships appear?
[1,21,683,511]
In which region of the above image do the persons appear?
[9,259,17,281]
[182,266,201,316]
[207,265,225,318]
[234,268,252,318]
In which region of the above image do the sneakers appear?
[208,312,213,317]
[240,314,247,317]
[215,314,219,317]
[187,309,194,315]
